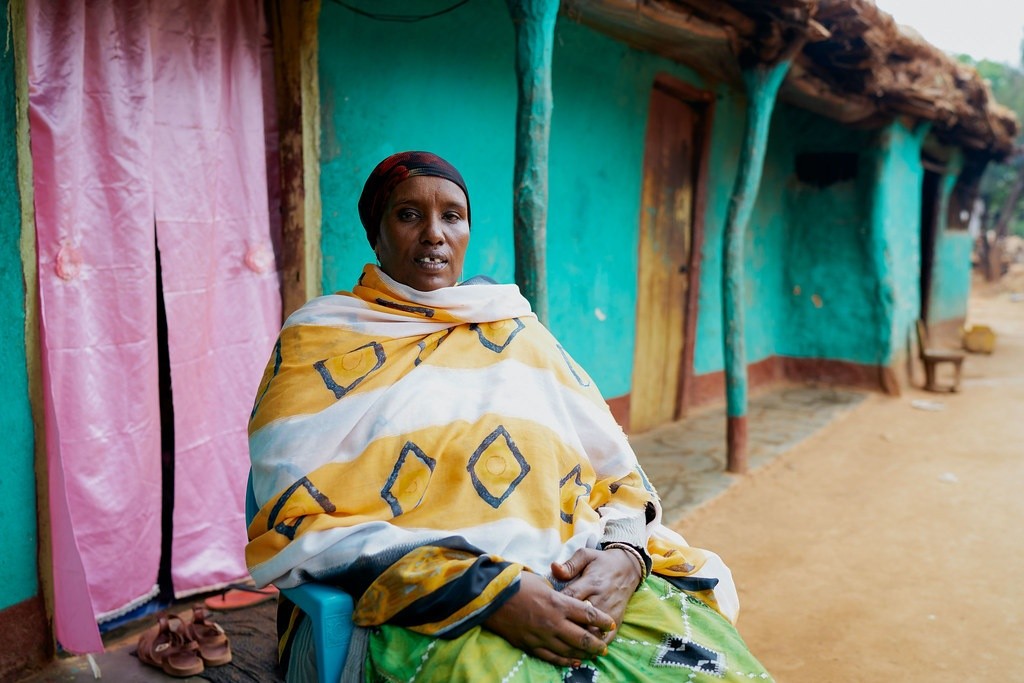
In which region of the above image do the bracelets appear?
[603,543,646,592]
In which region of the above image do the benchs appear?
[916,319,966,392]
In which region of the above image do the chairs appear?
[247,467,355,683]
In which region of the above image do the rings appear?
[583,600,593,606]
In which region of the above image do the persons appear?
[242,150,778,683]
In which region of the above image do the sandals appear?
[137,615,205,676]
[177,607,232,666]
